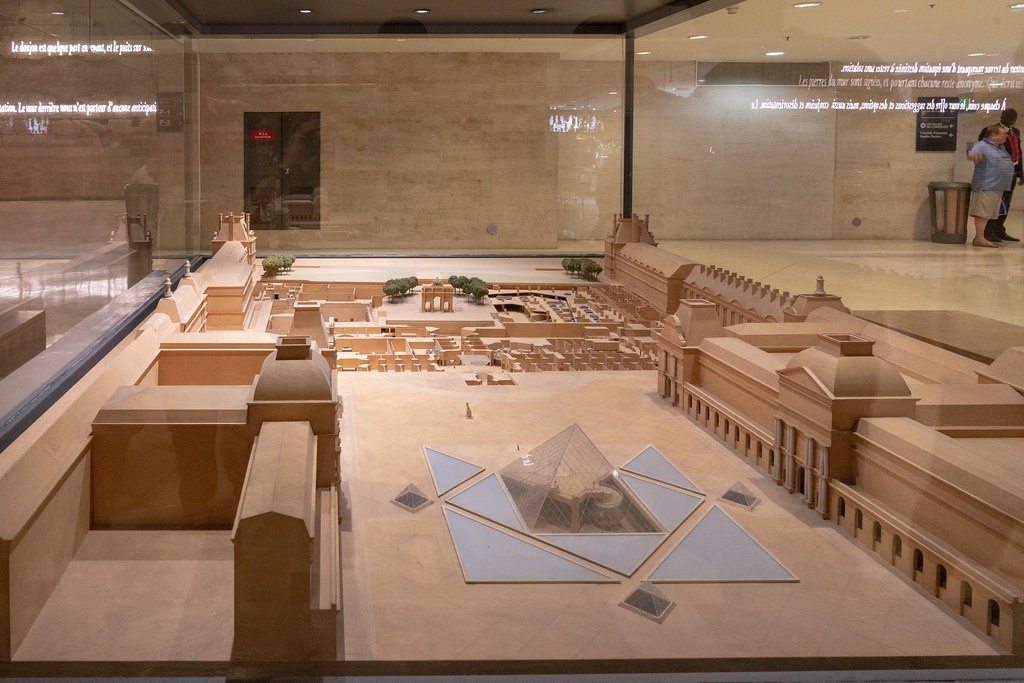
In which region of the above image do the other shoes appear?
[985,233,1002,242]
[972,239,999,248]
[996,232,1020,241]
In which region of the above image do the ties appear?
[1007,130,1018,162]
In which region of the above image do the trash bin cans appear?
[928,181,971,244]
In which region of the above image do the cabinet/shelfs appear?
[243,112,320,229]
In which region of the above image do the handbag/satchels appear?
[999,198,1008,215]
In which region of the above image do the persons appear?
[978,109,1024,241]
[968,126,1015,248]
